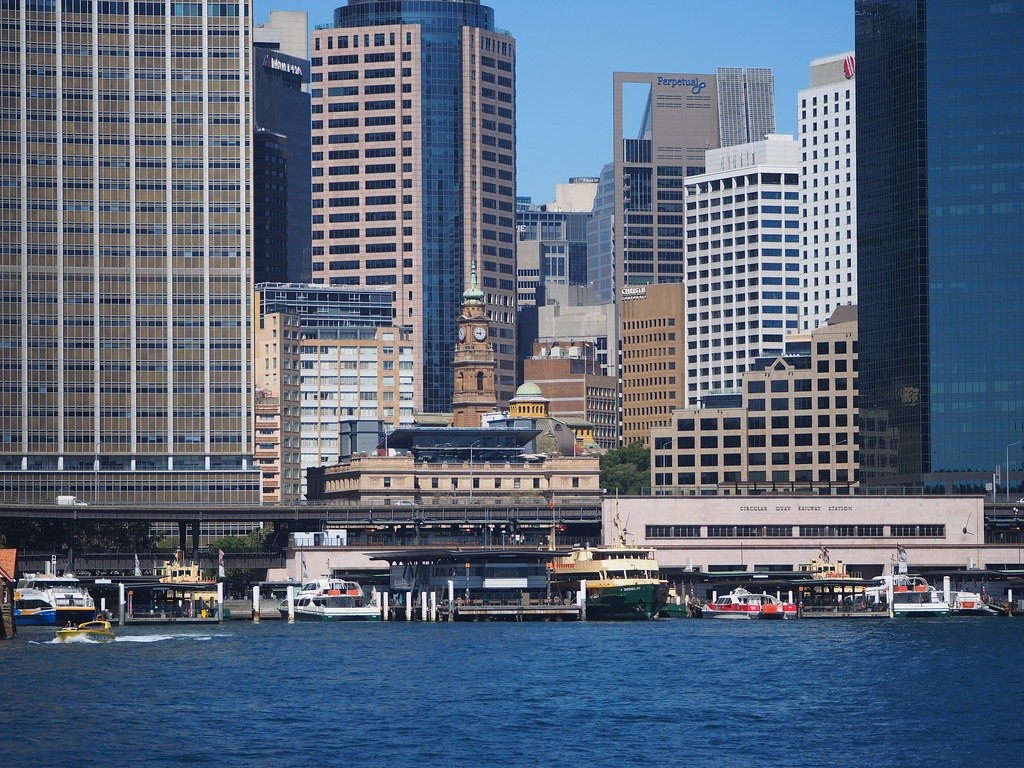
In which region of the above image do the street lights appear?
[663,437,677,496]
[469,439,481,504]
[831,439,847,496]
[1006,440,1022,503]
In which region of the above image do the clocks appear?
[458,327,467,342]
[473,325,487,341]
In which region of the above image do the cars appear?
[394,500,419,506]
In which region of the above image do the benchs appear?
[133,612,162,618]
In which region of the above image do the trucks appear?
[54,495,89,506]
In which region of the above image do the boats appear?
[13,572,97,626]
[54,621,116,643]
[276,575,381,621]
[701,586,798,621]
[794,540,1024,616]
[551,486,671,619]
